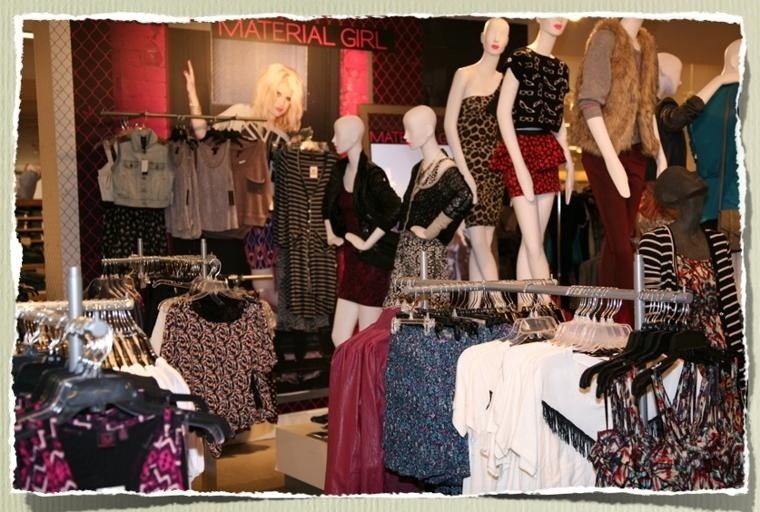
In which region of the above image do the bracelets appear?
[189,102,201,109]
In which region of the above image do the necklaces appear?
[415,150,444,183]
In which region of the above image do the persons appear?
[684,37,741,276]
[321,115,400,345]
[442,17,510,317]
[571,15,671,328]
[638,166,744,346]
[378,104,471,322]
[497,17,577,317]
[182,59,314,318]
[655,51,738,196]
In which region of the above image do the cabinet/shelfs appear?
[16,200,44,273]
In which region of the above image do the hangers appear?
[18,300,232,446]
[284,128,328,153]
[83,255,256,308]
[95,110,258,152]
